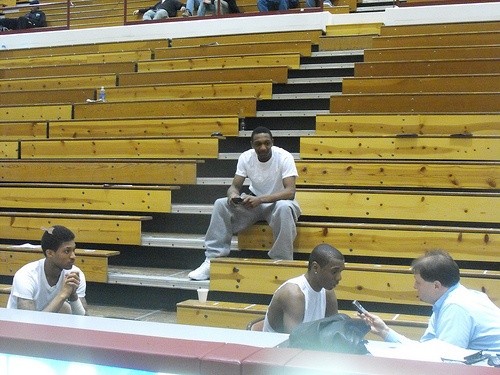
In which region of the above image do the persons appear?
[356,248,500,352]
[133,0,333,21]
[0,0,47,32]
[263,243,345,334]
[6,225,89,316]
[188,127,302,280]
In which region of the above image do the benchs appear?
[0,0,500,343]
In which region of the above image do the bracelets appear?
[71,293,77,296]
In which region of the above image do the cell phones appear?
[232,197,243,204]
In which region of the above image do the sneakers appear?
[188,257,210,280]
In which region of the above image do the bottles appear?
[99,85,106,100]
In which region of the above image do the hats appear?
[28,0,39,4]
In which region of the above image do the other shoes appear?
[182,10,191,17]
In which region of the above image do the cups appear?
[196,289,210,303]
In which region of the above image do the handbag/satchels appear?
[288,313,371,356]
